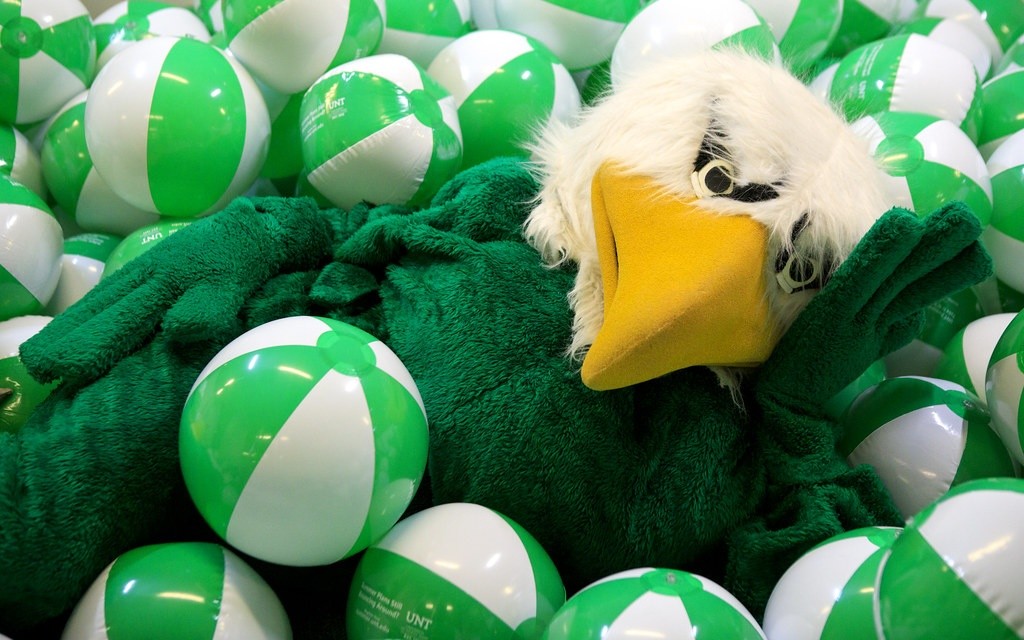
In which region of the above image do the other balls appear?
[0,1,1024,639]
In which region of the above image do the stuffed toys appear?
[1,37,994,640]
